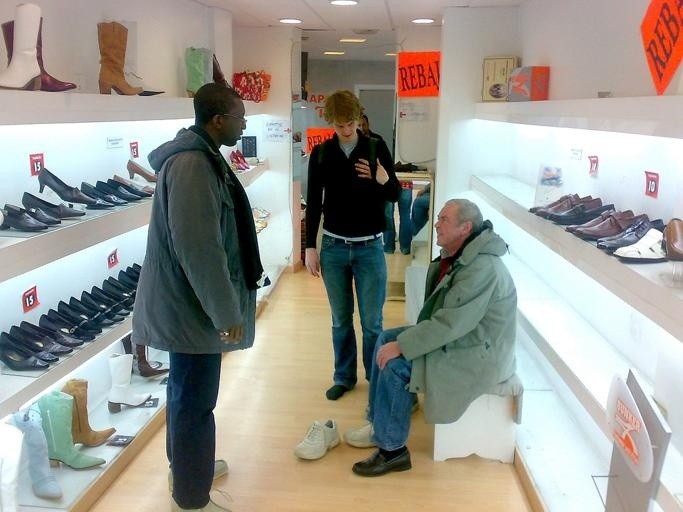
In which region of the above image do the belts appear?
[322,227,383,245]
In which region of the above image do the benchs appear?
[402,247,428,300]
[407,295,514,464]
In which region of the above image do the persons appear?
[357,113,384,140]
[129,83,264,512]
[303,90,402,400]
[343,198,518,477]
[410,182,430,241]
[383,182,411,255]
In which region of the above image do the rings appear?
[223,332,229,336]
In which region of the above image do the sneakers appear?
[168,458,227,492]
[345,422,385,447]
[294,419,341,460]
[170,492,233,512]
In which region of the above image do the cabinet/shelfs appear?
[292,97,312,265]
[0,85,292,512]
[459,91,680,512]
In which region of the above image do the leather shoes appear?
[353,448,412,476]
[0,320,94,372]
[3,159,158,233]
[229,149,270,235]
[528,193,683,262]
[41,262,168,332]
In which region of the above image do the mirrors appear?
[351,82,396,166]
[384,168,434,302]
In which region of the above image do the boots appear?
[96,21,145,95]
[0,3,76,92]
[12,405,62,500]
[107,352,151,412]
[37,390,106,470]
[185,46,243,100]
[61,379,117,448]
[121,332,169,378]
